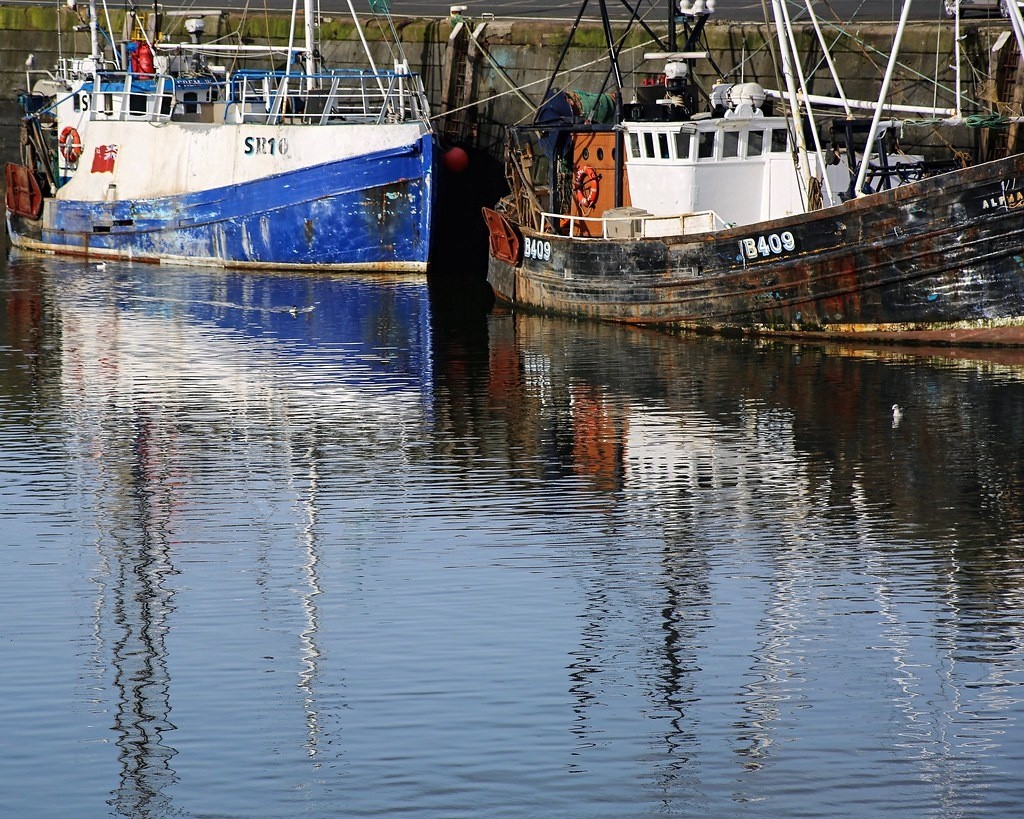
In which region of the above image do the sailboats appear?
[480,0,1024,350]
[3,0,440,279]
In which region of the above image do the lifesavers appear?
[573,167,597,205]
[59,127,81,161]
